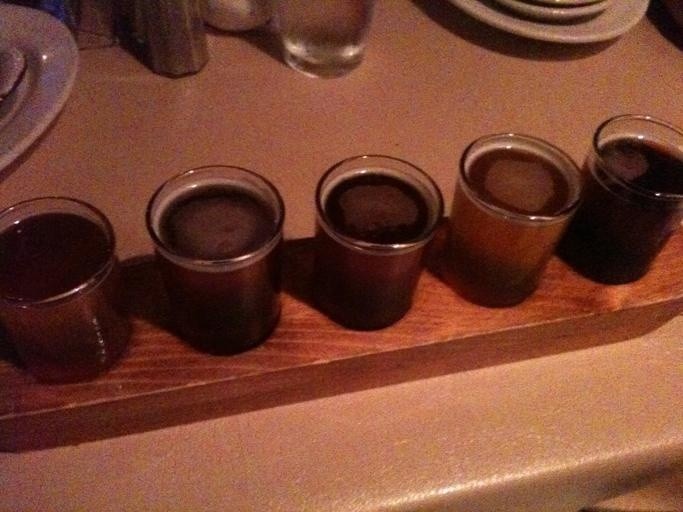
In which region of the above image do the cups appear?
[313,154,444,331]
[129,0,375,80]
[565,115,682,285]
[444,133,586,310]
[0,198,129,384]
[146,165,286,354]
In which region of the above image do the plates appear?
[450,0,655,45]
[0,3,79,175]
